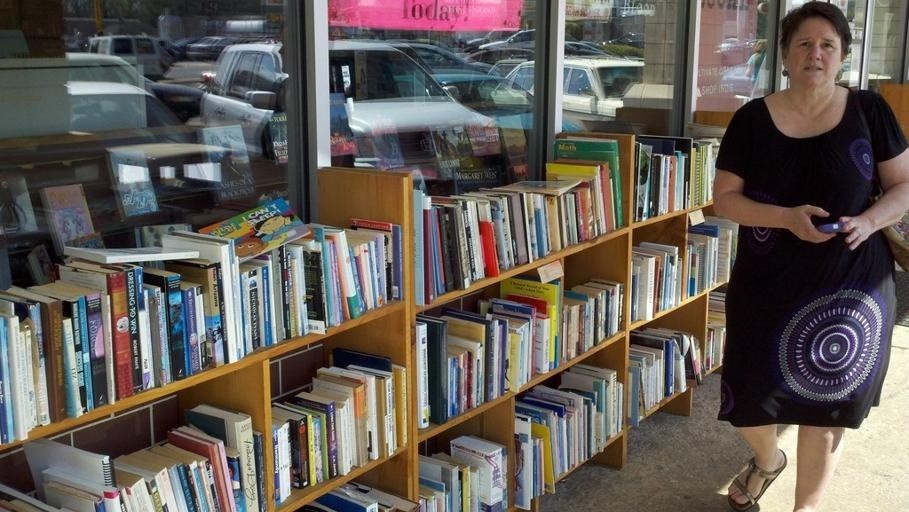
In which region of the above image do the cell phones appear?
[816,223,842,233]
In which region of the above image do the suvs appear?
[198,42,501,158]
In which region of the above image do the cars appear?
[1,78,247,249]
[68,14,769,146]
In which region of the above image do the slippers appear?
[728,448,787,512]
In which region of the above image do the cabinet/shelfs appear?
[0,108,747,512]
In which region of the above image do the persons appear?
[712,0,908,512]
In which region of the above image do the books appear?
[0,129,742,512]
